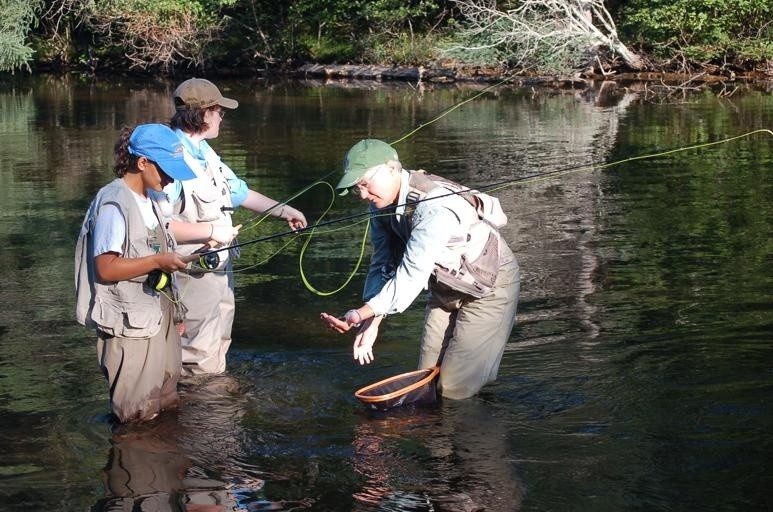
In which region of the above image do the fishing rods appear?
[183,43,570,269]
[146,126,768,291]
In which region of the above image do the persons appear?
[160,75,307,380]
[98,415,191,512]
[168,373,267,512]
[318,136,525,404]
[71,123,200,431]
[349,408,529,510]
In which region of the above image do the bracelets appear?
[278,204,285,218]
[350,309,362,327]
[209,223,214,240]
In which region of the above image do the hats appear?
[336,139,399,196]
[173,77,239,112]
[128,124,197,180]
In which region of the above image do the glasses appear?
[352,166,383,195]
[212,109,226,118]
